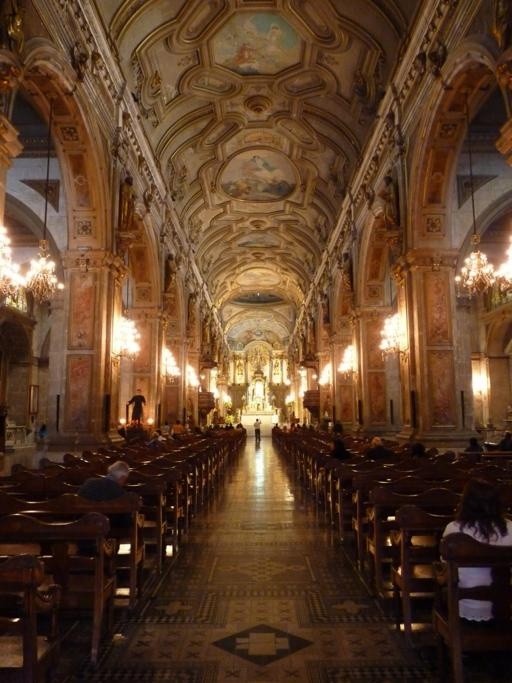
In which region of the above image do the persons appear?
[441,478,512,630]
[146,420,243,447]
[254,419,262,440]
[76,460,129,501]
[275,418,512,460]
[126,389,146,424]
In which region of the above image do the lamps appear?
[380,276,409,356]
[453,90,511,305]
[285,315,358,404]
[109,249,141,360]
[161,309,234,406]
[0,95,63,307]
